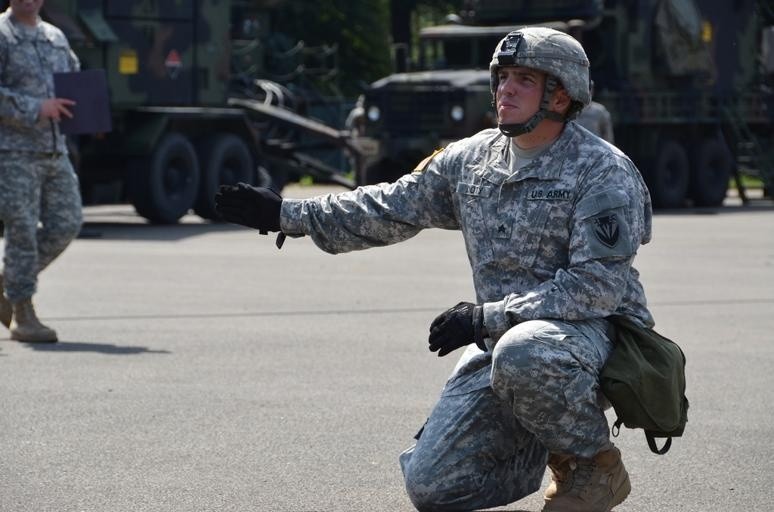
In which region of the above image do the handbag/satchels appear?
[598,318,688,438]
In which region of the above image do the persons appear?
[217,27,690,512]
[573,79,614,145]
[0,0,112,343]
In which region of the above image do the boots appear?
[9,300,57,343]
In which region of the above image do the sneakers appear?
[543,447,631,510]
[0,275,10,328]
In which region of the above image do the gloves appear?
[214,182,280,232]
[428,302,486,357]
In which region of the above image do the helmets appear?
[490,27,591,110]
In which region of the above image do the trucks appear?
[352,3,774,218]
